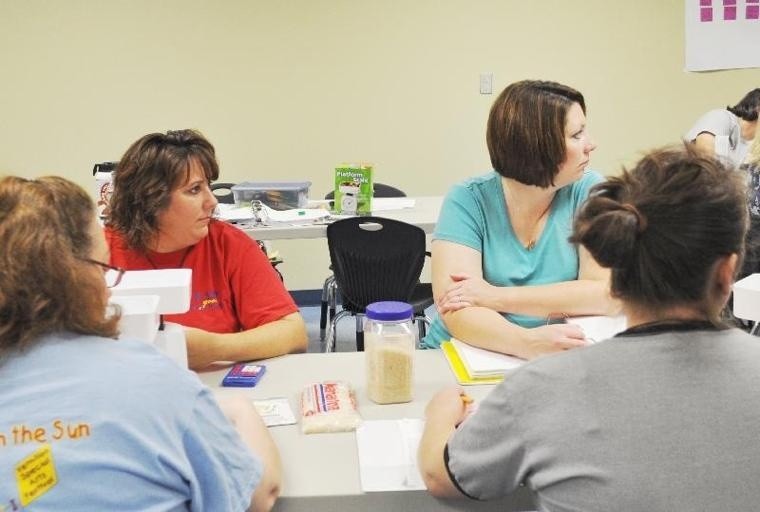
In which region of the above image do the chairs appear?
[320,184,407,352]
[325,216,434,352]
[210,183,283,282]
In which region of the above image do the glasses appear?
[80,258,124,288]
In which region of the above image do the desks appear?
[239,195,444,241]
[196,349,536,512]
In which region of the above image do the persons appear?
[680,85,760,272]
[1,170,285,512]
[422,79,647,366]
[416,131,760,510]
[87,126,311,374]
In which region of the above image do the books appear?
[210,199,331,229]
[438,335,529,385]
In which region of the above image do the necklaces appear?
[502,179,545,252]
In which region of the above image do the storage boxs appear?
[231,181,312,211]
